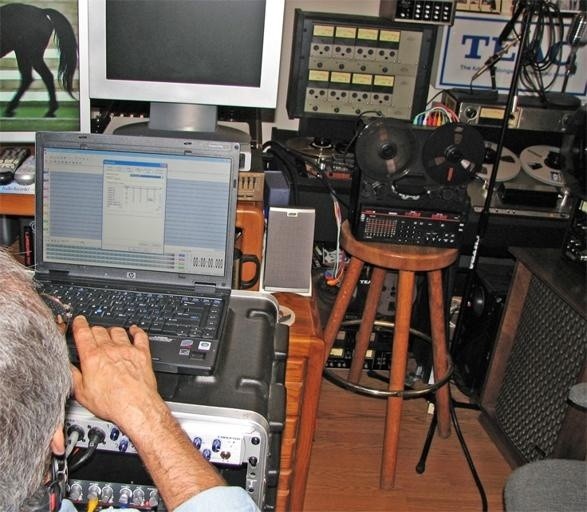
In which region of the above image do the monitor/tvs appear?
[88,0,287,144]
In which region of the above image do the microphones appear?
[472,35,522,80]
[544,11,587,107]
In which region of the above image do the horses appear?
[0,2,79,118]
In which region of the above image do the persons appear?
[0,245,262,512]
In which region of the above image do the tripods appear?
[367,0,535,474]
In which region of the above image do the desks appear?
[478,245,587,462]
[0,194,325,510]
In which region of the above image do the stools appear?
[321,216,459,492]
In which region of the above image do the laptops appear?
[32,131,240,374]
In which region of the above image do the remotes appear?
[14,154,36,185]
[0,147,31,185]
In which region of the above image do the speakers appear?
[259,207,315,298]
[450,262,515,396]
[271,146,358,254]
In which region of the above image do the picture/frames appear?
[0,0,91,143]
